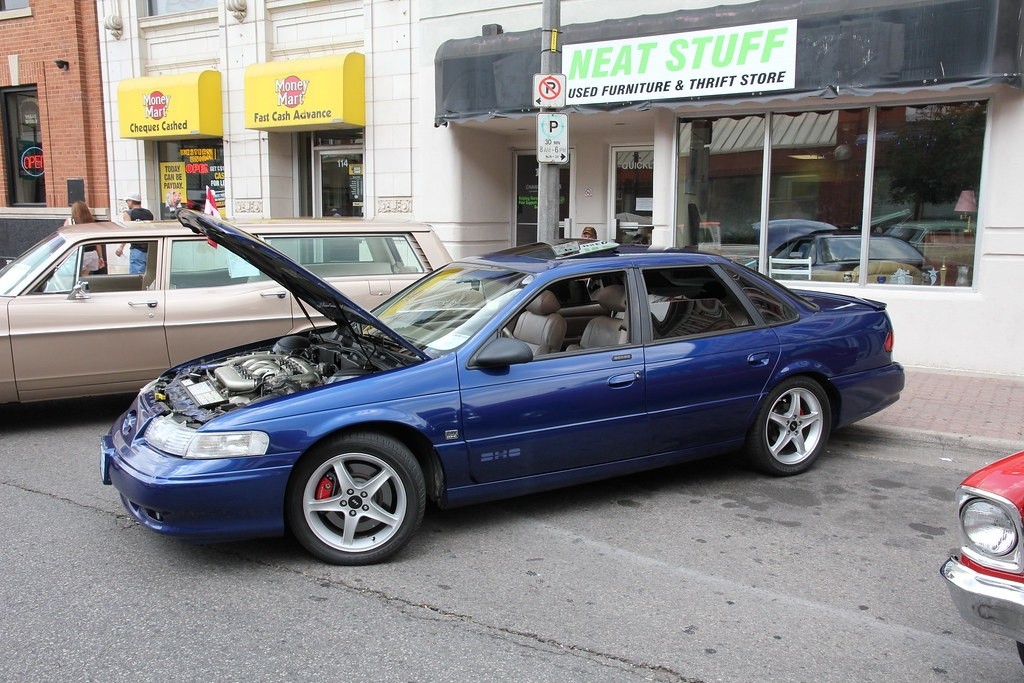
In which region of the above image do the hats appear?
[582,226,597,238]
[119,192,141,201]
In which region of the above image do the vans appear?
[0,219,453,406]
[885,222,977,255]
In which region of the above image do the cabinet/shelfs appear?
[923,231,975,268]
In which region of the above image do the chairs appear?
[565,285,630,355]
[512,290,566,357]
[768,255,812,281]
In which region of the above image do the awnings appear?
[434,0,1024,128]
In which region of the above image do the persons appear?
[581,226,598,240]
[115,192,153,274]
[631,234,648,244]
[64,200,105,275]
[331,208,341,216]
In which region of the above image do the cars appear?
[940,448,1023,665]
[751,219,839,254]
[744,233,935,284]
[101,207,904,566]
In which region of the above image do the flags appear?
[204,185,222,249]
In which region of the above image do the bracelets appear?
[99,256,103,259]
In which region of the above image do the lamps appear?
[952,190,977,233]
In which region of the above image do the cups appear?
[843,271,857,282]
